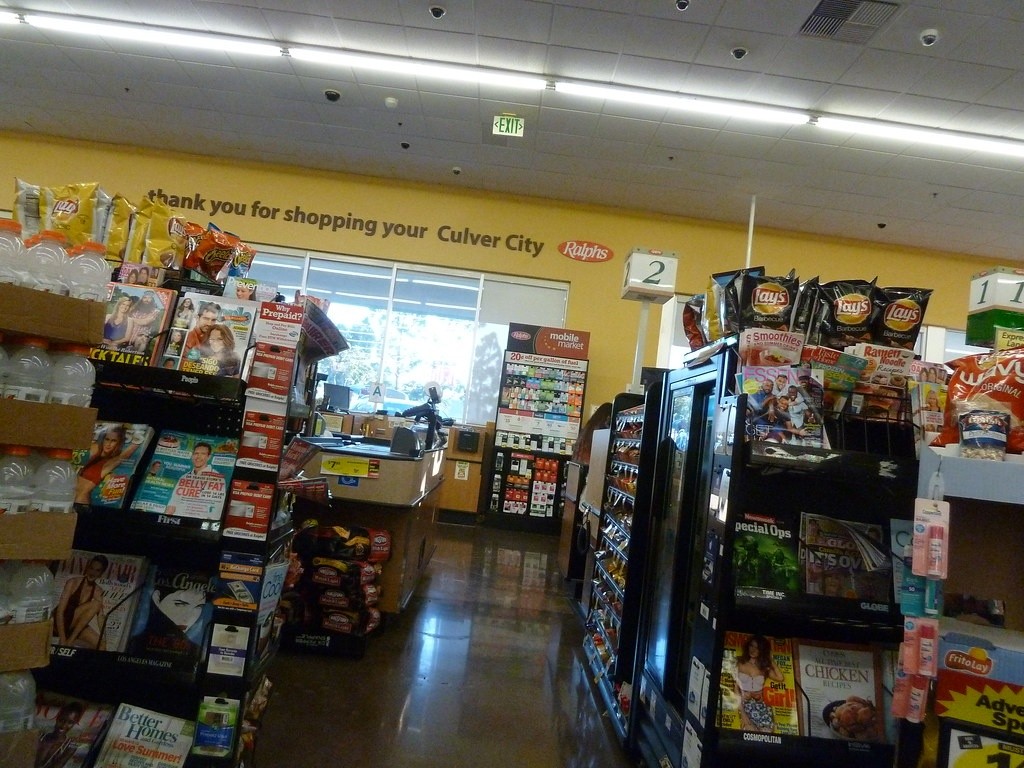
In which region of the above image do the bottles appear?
[0,214,110,303]
[1,671,35,732]
[0,444,77,517]
[1,561,56,624]
[2,332,96,408]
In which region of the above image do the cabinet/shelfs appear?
[0,279,1024,767]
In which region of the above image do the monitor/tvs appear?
[324,383,350,411]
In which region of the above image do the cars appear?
[349,384,408,403]
[353,395,446,427]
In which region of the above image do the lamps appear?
[0,5,1022,159]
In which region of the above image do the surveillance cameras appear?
[920,29,938,46]
